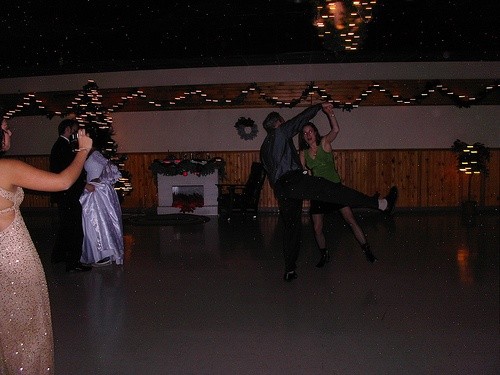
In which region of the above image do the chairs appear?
[214,161,267,233]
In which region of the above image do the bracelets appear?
[328,114,335,117]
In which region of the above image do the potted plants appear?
[451,139,492,229]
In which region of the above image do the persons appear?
[0,109,93,374]
[297,106,379,269]
[50,119,87,258]
[259,102,399,282]
[77,125,126,268]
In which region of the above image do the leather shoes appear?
[315,248,330,268]
[65,263,92,273]
[362,244,378,265]
[283,271,298,282]
[383,186,397,215]
